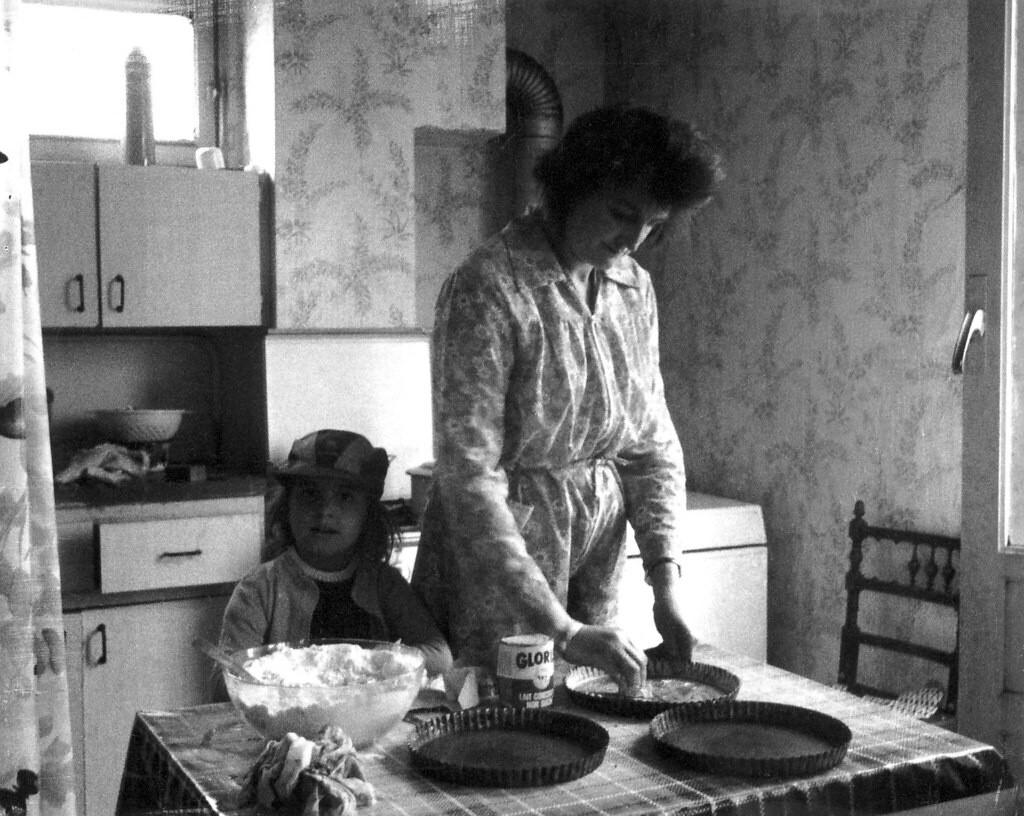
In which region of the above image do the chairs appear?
[834,499,960,733]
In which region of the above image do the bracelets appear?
[643,558,682,586]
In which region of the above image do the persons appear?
[209,430,452,704]
[411,106,726,698]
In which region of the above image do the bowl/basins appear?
[221,636,425,751]
[91,409,189,443]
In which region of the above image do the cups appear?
[498,634,556,709]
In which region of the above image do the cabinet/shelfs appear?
[29,159,271,338]
[55,508,265,816]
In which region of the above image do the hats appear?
[270,429,389,498]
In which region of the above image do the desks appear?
[113,642,1015,816]
